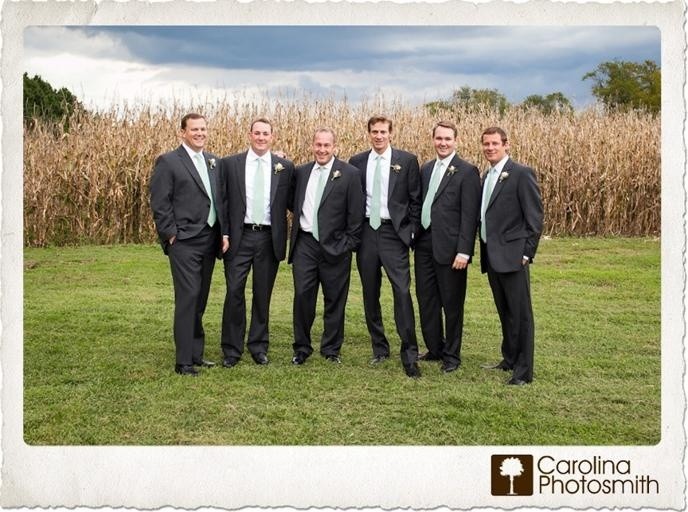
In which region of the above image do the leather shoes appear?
[327,355,341,364]
[406,367,422,378]
[201,359,215,367]
[480,360,503,371]
[371,355,386,364]
[420,351,440,361]
[175,364,198,377]
[222,356,238,367]
[252,352,268,364]
[292,351,308,364]
[506,378,526,385]
[440,361,458,373]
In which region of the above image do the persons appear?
[222,118,295,369]
[411,121,483,372]
[288,128,364,365]
[149,113,228,377]
[478,126,544,386]
[348,115,421,378]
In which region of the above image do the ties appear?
[480,169,496,241]
[370,156,382,231]
[252,158,265,225]
[194,153,216,228]
[421,161,443,230]
[313,167,326,242]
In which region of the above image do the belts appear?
[366,218,392,224]
[244,224,271,231]
